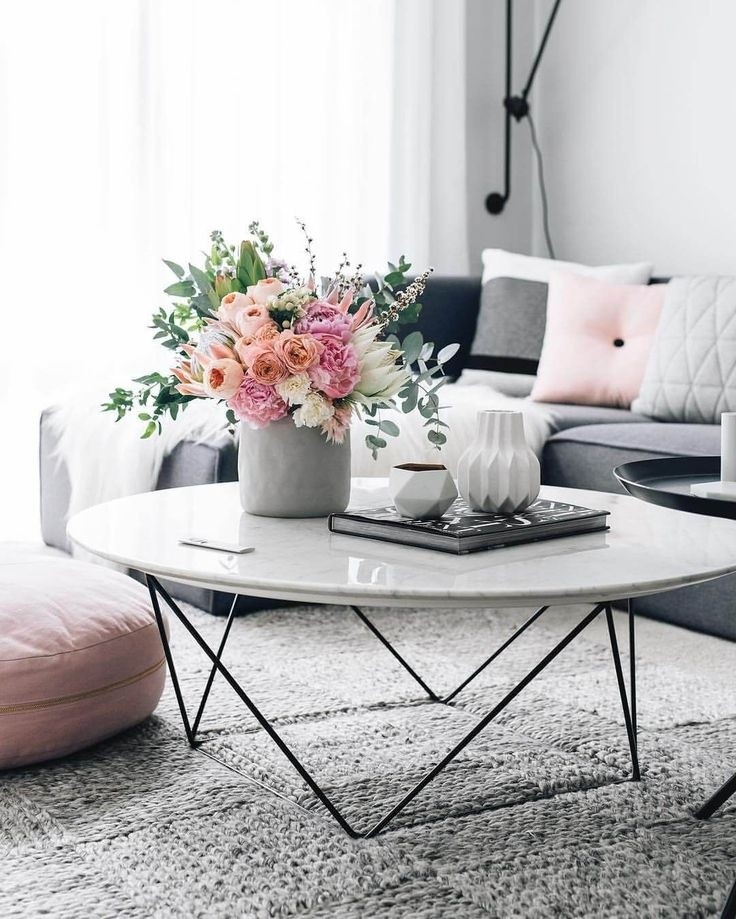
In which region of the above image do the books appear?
[327,495,611,557]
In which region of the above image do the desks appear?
[67,480,736,840]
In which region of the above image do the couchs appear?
[36,377,735,641]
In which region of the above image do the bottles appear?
[457,410,541,515]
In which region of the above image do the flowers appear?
[97,216,461,464]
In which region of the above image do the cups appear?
[389,464,459,520]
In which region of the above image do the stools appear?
[0,552,170,767]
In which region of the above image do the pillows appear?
[630,276,736,425]
[450,247,653,398]
[322,276,481,385]
[523,271,667,409]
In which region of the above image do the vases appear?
[237,414,351,519]
